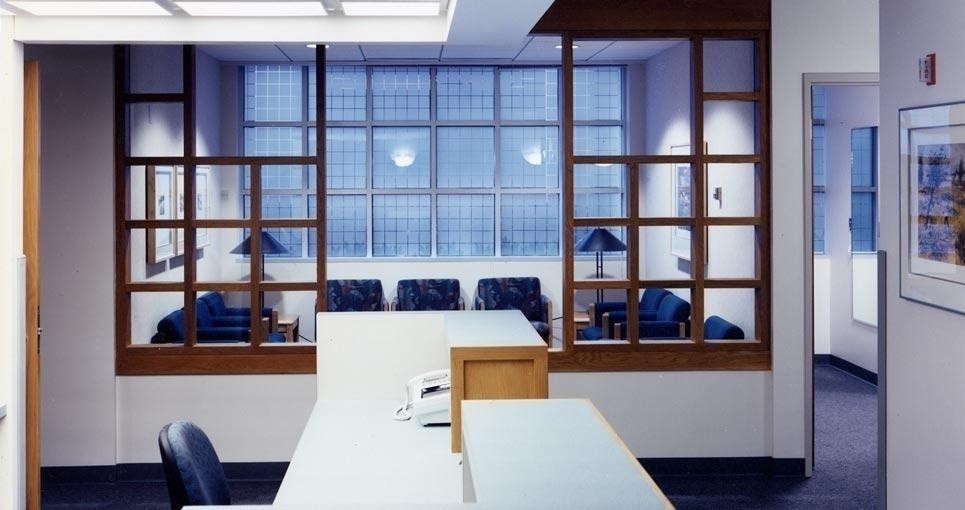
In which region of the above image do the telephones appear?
[407,369,451,426]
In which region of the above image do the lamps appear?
[574,226,627,301]
[230,233,288,311]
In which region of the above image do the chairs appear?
[391,277,466,312]
[471,276,553,348]
[148,292,283,344]
[157,420,235,509]
[318,276,389,313]
[580,288,744,339]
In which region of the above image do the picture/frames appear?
[896,101,965,315]
[145,164,212,265]
[668,141,709,264]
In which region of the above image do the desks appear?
[266,311,679,510]
